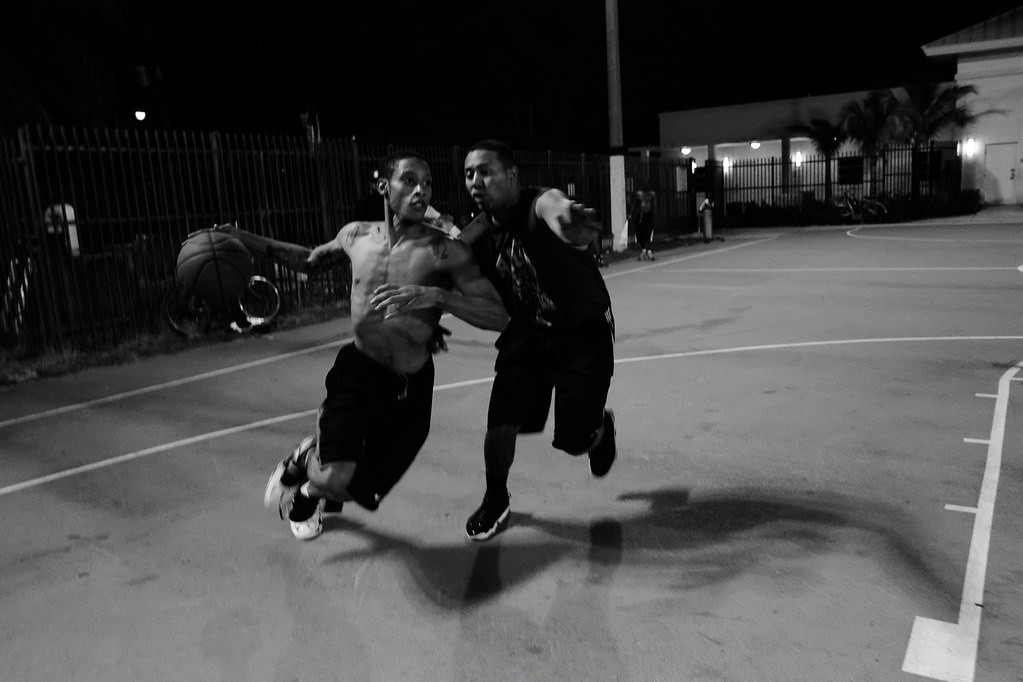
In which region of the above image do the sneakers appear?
[263,435,317,518]
[465,489,512,541]
[285,481,326,540]
[589,406,616,478]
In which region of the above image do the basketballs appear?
[176,231,253,301]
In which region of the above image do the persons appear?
[453,142,617,542]
[180,153,507,542]
[636,179,658,260]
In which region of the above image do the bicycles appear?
[828,185,888,225]
[161,274,282,338]
